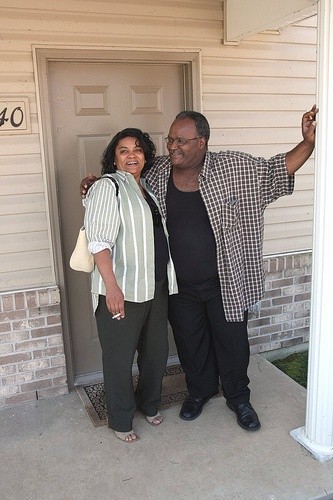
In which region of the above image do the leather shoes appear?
[226,399,261,431]
[180,395,211,420]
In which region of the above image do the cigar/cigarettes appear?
[112,312,122,319]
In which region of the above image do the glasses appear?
[164,137,201,146]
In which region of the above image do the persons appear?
[82,128,179,444]
[80,105,319,431]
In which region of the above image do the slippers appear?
[145,411,163,427]
[114,430,139,442]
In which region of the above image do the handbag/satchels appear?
[70,176,119,273]
